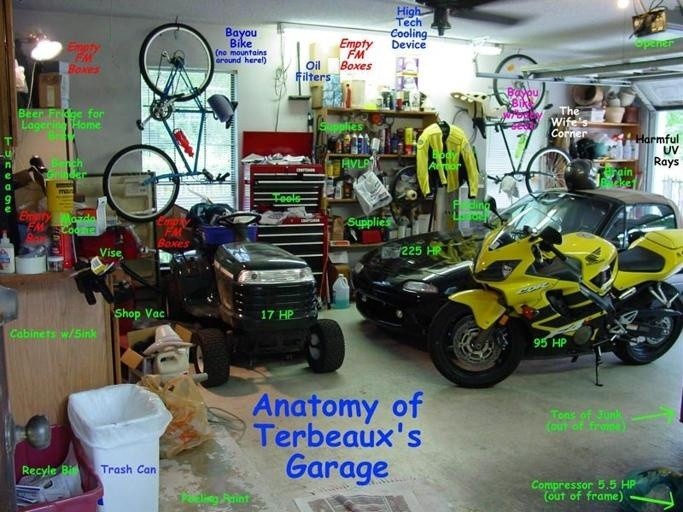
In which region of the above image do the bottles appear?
[334,182,342,200]
[344,84,351,108]
[357,131,363,153]
[374,85,409,112]
[386,127,391,154]
[614,139,623,161]
[364,130,370,154]
[343,175,351,197]
[335,137,342,153]
[326,159,333,176]
[350,130,357,155]
[629,138,639,162]
[342,132,350,153]
[411,139,416,153]
[397,141,403,154]
[391,140,397,154]
[378,128,385,154]
[622,139,631,160]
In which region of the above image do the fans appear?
[391,0,525,37]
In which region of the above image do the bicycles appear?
[102,23,238,222]
[473,54,574,205]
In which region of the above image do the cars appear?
[350,187,683,343]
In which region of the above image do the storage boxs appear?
[154,204,197,255]
[201,224,258,264]
[70,196,106,236]
[39,73,70,108]
[574,106,605,122]
[120,324,192,384]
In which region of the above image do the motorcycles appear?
[429,196,680,388]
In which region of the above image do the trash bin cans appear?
[67,383,174,512]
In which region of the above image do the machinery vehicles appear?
[166,203,345,387]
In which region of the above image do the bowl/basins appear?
[14,255,47,276]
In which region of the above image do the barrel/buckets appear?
[332,273,351,309]
[154,350,191,375]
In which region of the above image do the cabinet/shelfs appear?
[314,106,441,300]
[549,122,641,187]
[11,106,76,198]
[0,262,122,432]
[0,0,19,154]
[239,130,329,309]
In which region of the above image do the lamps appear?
[15,29,63,61]
